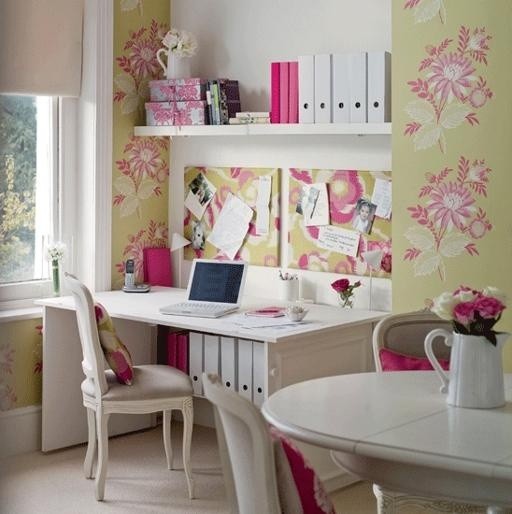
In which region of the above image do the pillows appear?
[94,301,134,385]
[378,346,449,371]
[274,434,337,512]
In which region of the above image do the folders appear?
[189,331,267,409]
[298,49,391,123]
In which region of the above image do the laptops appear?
[159,258,249,319]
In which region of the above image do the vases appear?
[338,293,356,308]
[423,328,510,409]
[156,48,191,78]
[52,260,60,296]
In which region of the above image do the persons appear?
[353,201,371,233]
[192,180,208,204]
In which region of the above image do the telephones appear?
[122,258,151,293]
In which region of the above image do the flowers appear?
[430,283,507,347]
[160,28,199,58]
[45,240,66,291]
[331,277,362,308]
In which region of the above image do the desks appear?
[32,284,392,462]
[260,371,512,513]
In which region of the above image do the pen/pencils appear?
[278,269,299,280]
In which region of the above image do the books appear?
[245,305,286,318]
[206,61,298,124]
[143,246,173,287]
[164,331,265,410]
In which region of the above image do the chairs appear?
[372,310,448,371]
[64,272,197,502]
[199,371,282,514]
